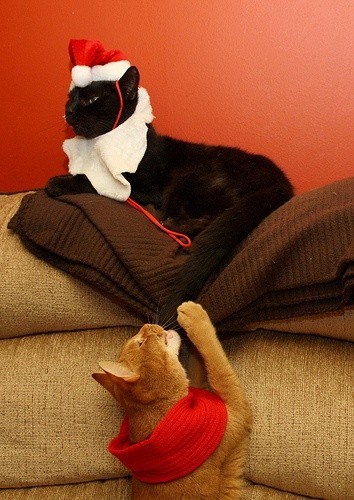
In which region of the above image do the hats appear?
[70,39,131,91]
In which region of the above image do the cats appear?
[91,301,253,500]
[44,65,295,370]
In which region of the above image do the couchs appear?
[0,176,354,500]
